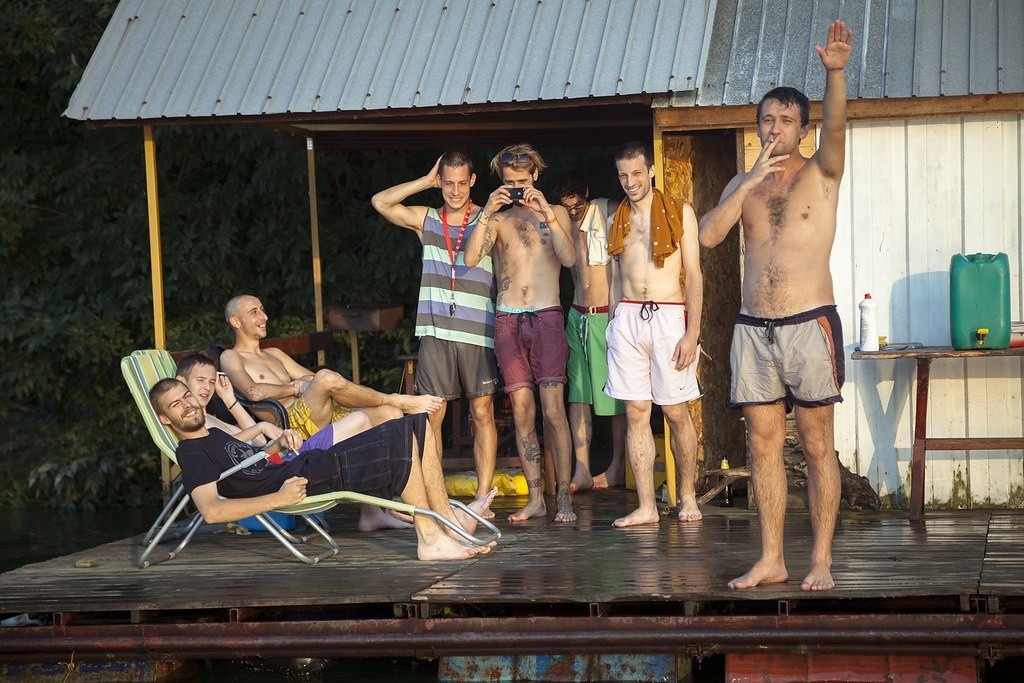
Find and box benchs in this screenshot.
[168,330,339,430]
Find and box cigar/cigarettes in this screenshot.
[218,372,226,375]
[293,448,299,456]
[770,139,773,142]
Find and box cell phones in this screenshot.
[502,187,525,199]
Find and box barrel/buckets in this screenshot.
[950,252,1010,349]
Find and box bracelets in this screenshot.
[478,219,486,225]
[228,400,238,411]
[547,217,556,224]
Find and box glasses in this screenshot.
[501,152,536,165]
[564,199,586,212]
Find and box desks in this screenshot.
[851,346,1024,524]
[400,351,545,469]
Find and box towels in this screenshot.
[608,188,686,268]
[578,197,612,265]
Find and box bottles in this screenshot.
[859,294,879,352]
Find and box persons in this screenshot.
[698,19,850,589]
[219,295,442,522]
[178,353,497,536]
[609,143,703,528]
[372,152,499,518]
[551,170,628,491]
[463,145,576,521]
[152,379,497,560]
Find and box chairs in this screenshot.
[119,348,500,568]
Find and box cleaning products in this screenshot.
[859,292,879,353]
